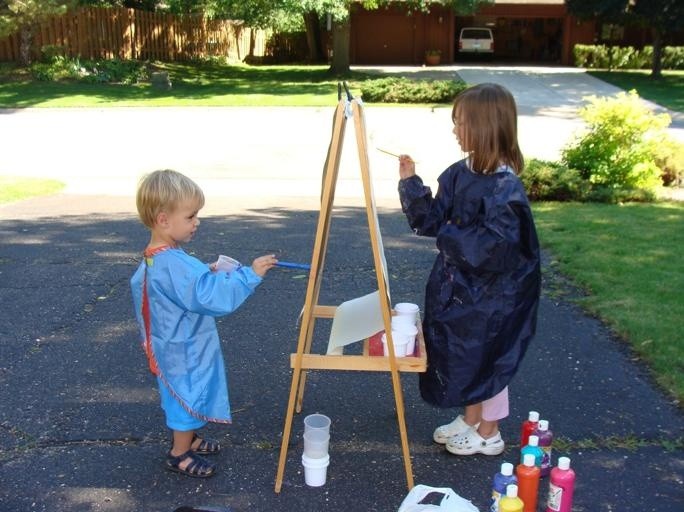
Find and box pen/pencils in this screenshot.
[277,261,311,270]
[378,148,419,166]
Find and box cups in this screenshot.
[213,255,242,272]
[380,302,419,357]
[301,412,333,488]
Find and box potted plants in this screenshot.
[425,48,442,65]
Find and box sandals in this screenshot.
[444,427,506,457]
[432,413,480,444]
[162,445,215,479]
[190,434,221,455]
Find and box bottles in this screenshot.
[489,410,575,512]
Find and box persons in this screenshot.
[397,82,542,457]
[129,169,278,479]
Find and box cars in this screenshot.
[458,27,495,59]
